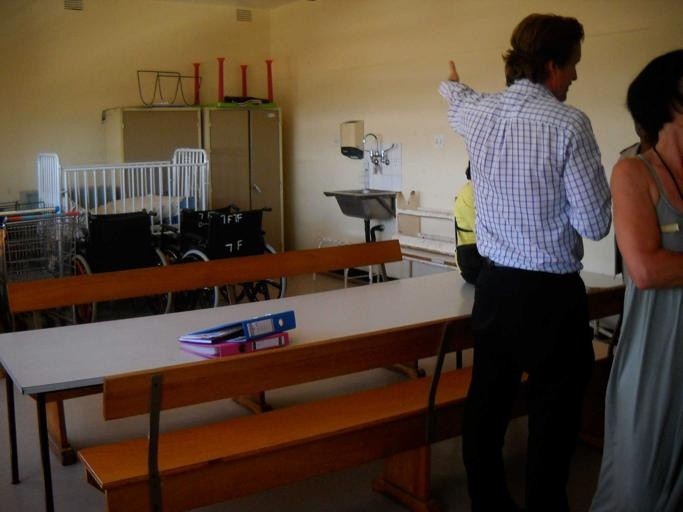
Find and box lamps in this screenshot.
[359,132,396,169]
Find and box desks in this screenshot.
[1,270,629,510]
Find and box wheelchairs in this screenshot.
[54,204,288,328]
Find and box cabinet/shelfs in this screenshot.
[392,190,460,278]
[103,108,286,255]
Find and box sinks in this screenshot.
[323,188,395,220]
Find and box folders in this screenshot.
[176,310,297,360]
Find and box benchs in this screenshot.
[7,241,426,467]
[78,286,628,511]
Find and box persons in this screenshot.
[614,117,653,284]
[586,48,683,512]
[436,12,612,512]
[452,159,481,285]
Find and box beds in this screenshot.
[34,149,210,272]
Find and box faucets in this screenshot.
[362,133,379,158]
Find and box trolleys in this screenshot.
[0,200,81,331]
